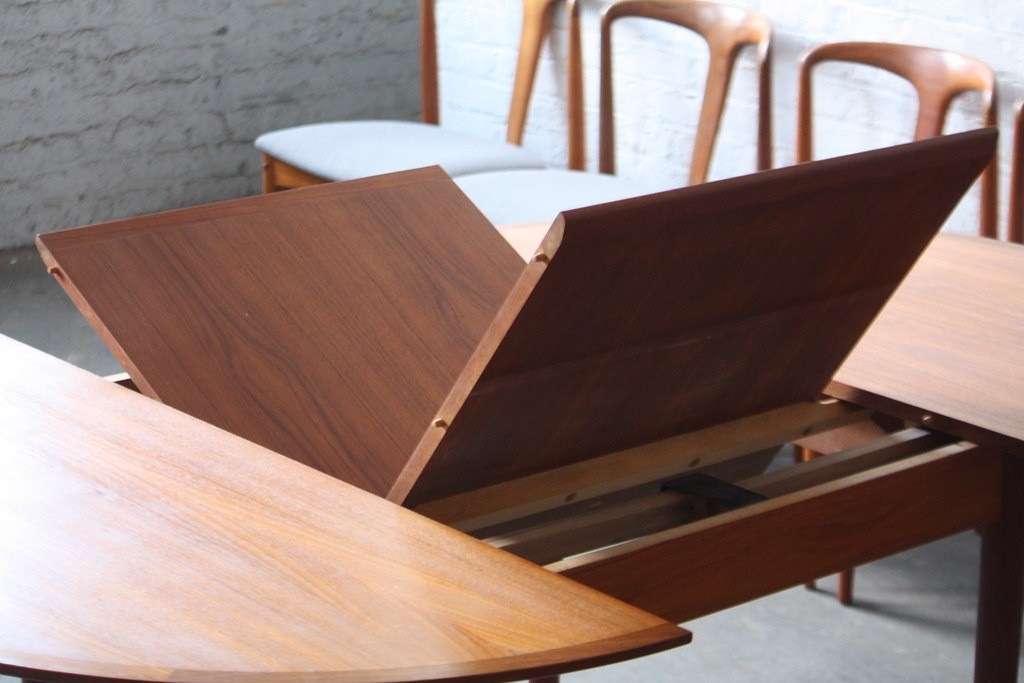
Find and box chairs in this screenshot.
[794,44,1024,247]
[248,0,580,195]
[450,1,778,264]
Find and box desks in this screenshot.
[0,129,1024,682]
